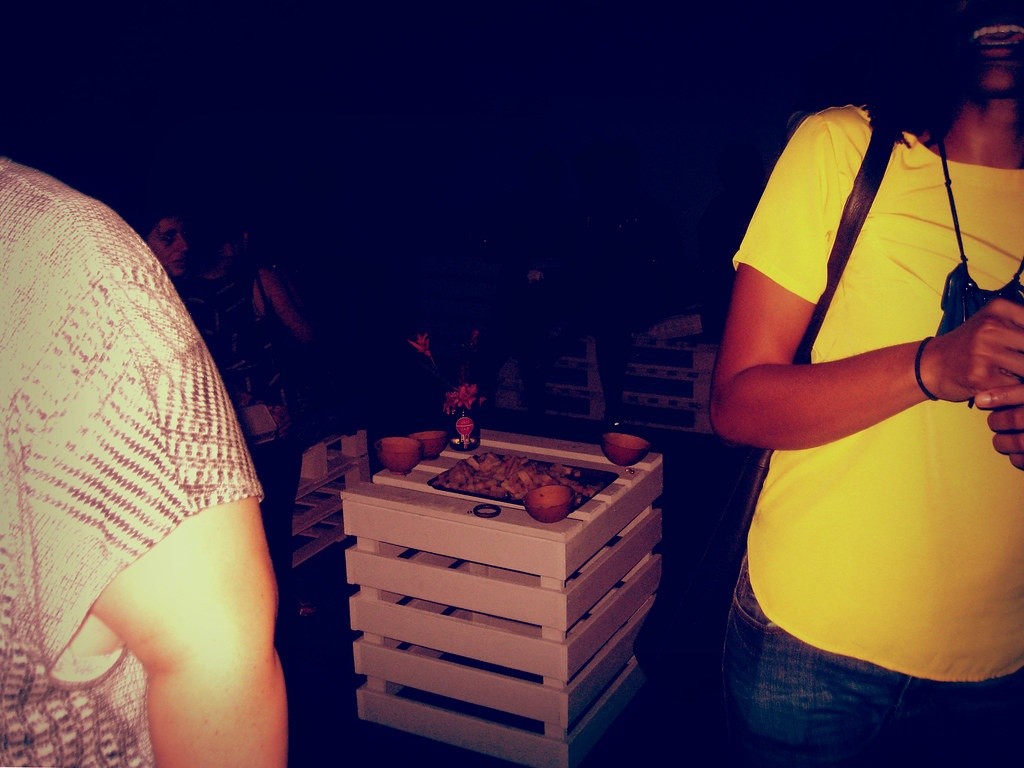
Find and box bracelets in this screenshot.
[474,504,501,517]
[914,337,938,402]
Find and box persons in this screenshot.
[710,1,1024,767]
[0,157,291,767]
[131,196,313,686]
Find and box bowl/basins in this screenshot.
[375,430,447,475]
[524,486,573,524]
[600,433,650,466]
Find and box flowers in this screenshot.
[407,330,487,414]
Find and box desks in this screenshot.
[290,431,369,570]
[340,427,663,768]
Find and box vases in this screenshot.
[447,409,482,451]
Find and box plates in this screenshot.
[427,454,619,518]
